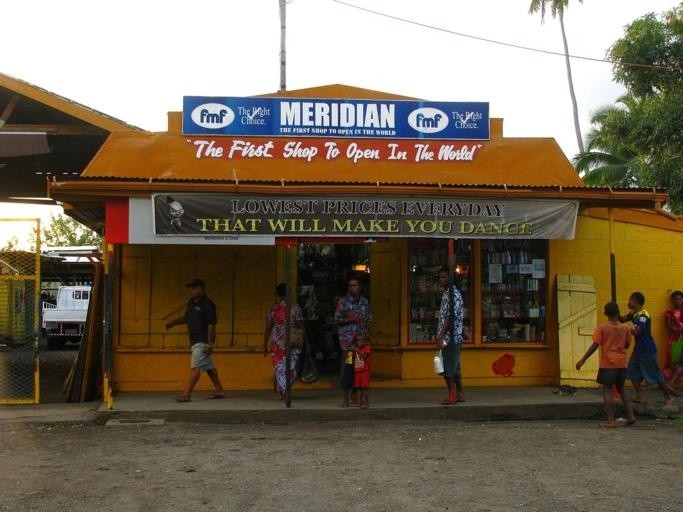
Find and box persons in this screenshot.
[262,282,306,400]
[166,278,226,403]
[299,268,322,384]
[333,277,373,408]
[563,291,683,429]
[435,267,466,404]
[345,335,373,409]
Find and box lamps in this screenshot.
[351,257,366,270]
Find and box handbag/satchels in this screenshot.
[284,327,304,349]
[671,341,683,365]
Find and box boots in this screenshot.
[442,372,465,405]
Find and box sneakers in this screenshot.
[276,390,287,400]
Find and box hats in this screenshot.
[187,280,206,287]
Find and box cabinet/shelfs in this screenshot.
[409,245,471,342]
[483,249,545,342]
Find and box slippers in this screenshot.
[204,393,226,399]
[176,395,192,401]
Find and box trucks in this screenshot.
[41,284,91,348]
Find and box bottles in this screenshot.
[486,244,528,264]
[540,304,545,317]
[530,326,536,341]
[434,356,444,374]
